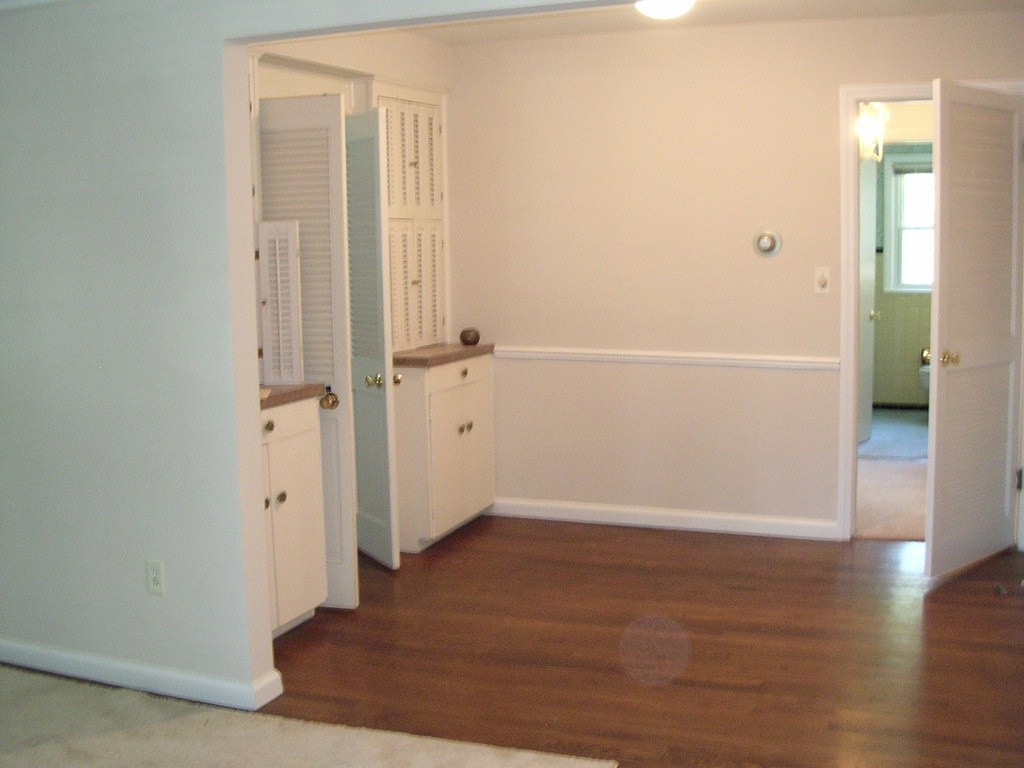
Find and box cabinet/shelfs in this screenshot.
[260,397,329,641]
[397,352,497,554]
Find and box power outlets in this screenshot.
[145,559,163,593]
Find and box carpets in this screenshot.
[854,453,927,543]
[0,663,621,768]
